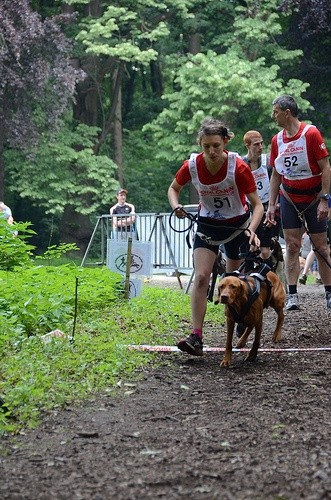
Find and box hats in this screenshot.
[116,187,128,195]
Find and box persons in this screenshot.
[0,200,18,239]
[241,130,284,272]
[168,120,264,356]
[267,95,331,310]
[110,188,139,241]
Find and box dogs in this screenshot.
[214,271,286,366]
[207,250,226,302]
[254,234,312,294]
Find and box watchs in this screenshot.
[321,194,330,200]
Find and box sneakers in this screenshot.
[299,273,307,285]
[236,323,247,338]
[315,277,323,284]
[178,333,204,356]
[325,290,331,308]
[285,291,300,310]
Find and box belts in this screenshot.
[282,183,322,196]
